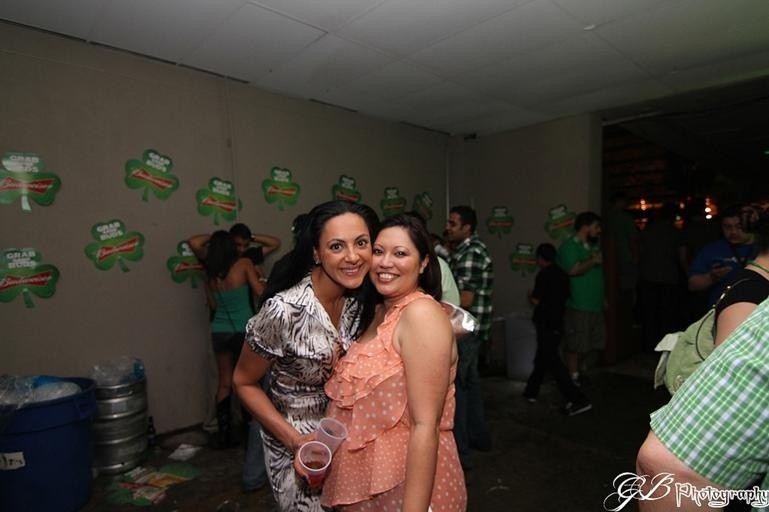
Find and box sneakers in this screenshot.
[523,392,536,403]
[203,415,219,433]
[567,400,592,417]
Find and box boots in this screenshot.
[215,393,240,450]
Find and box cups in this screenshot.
[296,417,348,488]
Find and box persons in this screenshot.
[295,212,468,511]
[634,294,769,511]
[230,198,384,512]
[188,201,769,458]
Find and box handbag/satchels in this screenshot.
[664,308,716,395]
